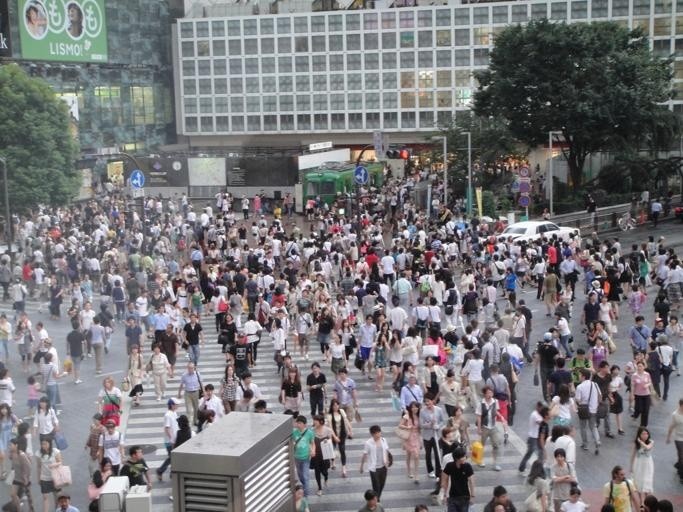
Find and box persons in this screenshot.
[68,4,83,37]
[26,7,48,37]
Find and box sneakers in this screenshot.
[315,464,347,496]
[490,463,527,479]
[579,429,626,455]
[155,468,163,481]
[131,393,166,409]
[72,370,102,384]
[407,469,442,484]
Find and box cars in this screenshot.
[495,220,582,247]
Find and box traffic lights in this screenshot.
[386,148,410,160]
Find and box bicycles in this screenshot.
[617,211,638,232]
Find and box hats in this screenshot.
[592,280,601,288]
[93,413,115,428]
[56,493,71,500]
[654,318,664,324]
[542,325,560,342]
[176,414,190,427]
[658,334,668,343]
[165,397,182,405]
[447,325,456,333]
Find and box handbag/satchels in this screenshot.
[532,369,540,387]
[620,271,630,283]
[49,466,72,489]
[444,305,454,315]
[422,344,439,358]
[415,318,433,331]
[470,441,484,466]
[62,353,74,376]
[198,388,204,399]
[352,406,362,423]
[350,335,363,370]
[498,268,506,275]
[54,433,70,452]
[217,330,228,345]
[660,363,673,375]
[121,375,131,392]
[218,299,230,312]
[146,363,154,372]
[445,289,458,305]
[524,491,544,512]
[400,346,417,357]
[570,480,578,488]
[507,406,516,427]
[319,438,335,460]
[420,275,432,294]
[393,426,412,440]
[577,402,609,419]
[181,340,189,351]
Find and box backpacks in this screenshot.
[289,254,302,271]
[593,289,604,303]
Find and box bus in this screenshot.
[301,158,386,216]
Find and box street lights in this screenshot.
[549,130,564,216]
[80,152,147,254]
[460,132,474,219]
[431,135,449,208]
